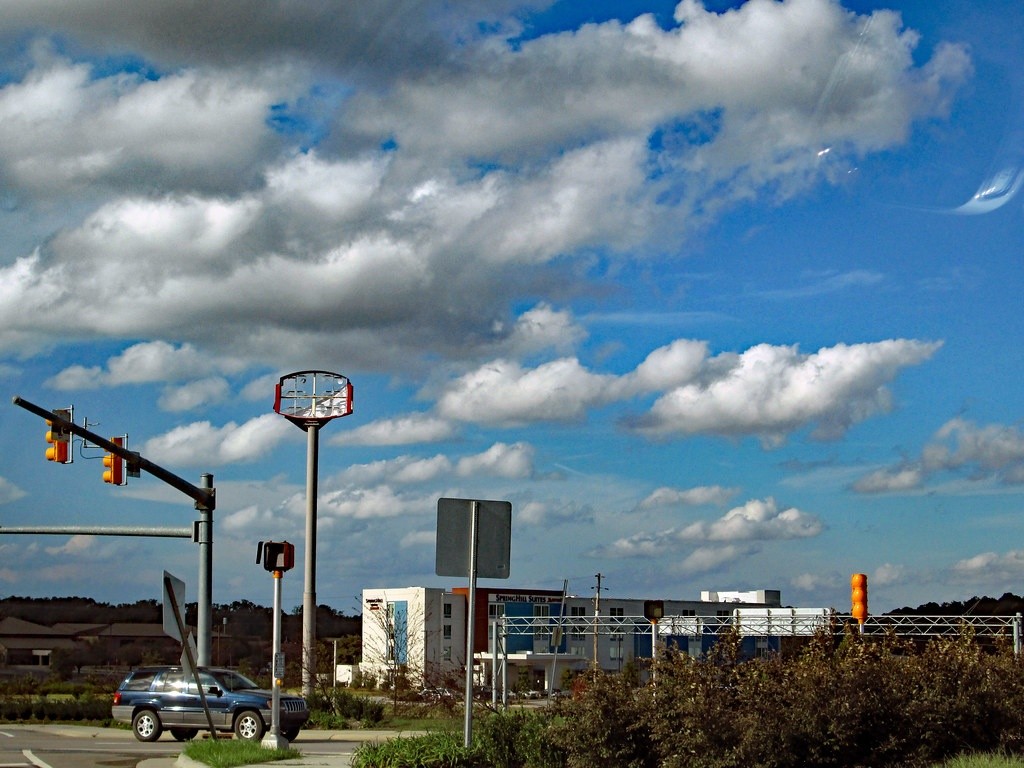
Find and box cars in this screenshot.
[475,686,573,701]
[416,687,465,702]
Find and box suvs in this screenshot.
[111,664,311,743]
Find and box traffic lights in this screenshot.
[643,600,664,624]
[45,409,72,463]
[262,541,290,571]
[101,438,122,486]
